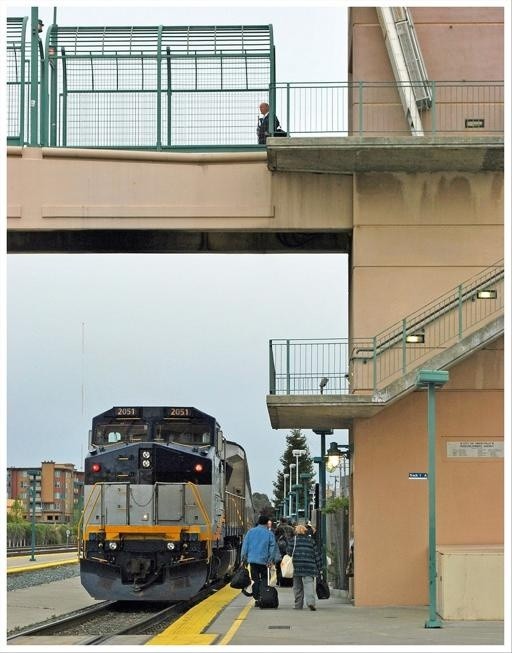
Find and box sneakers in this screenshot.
[308,604,317,611]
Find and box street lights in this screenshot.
[319,376,329,581]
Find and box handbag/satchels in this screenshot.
[231,569,250,589]
[316,573,330,599]
[279,555,293,587]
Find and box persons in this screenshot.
[240,515,276,586]
[275,516,293,541]
[305,524,313,535]
[256,101,280,144]
[288,524,322,610]
[267,519,275,532]
[289,522,292,525]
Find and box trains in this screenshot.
[76,405,257,602]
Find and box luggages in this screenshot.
[259,567,279,609]
